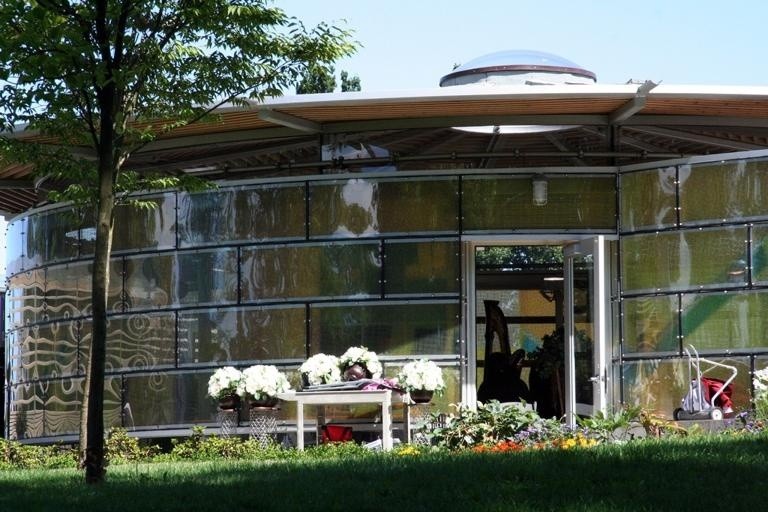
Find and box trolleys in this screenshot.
[673,343,738,420]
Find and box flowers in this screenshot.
[397,356,449,397]
[209,343,384,401]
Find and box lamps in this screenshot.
[531,173,551,206]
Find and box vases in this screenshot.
[217,393,278,408]
[408,388,434,403]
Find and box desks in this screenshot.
[295,390,411,457]
